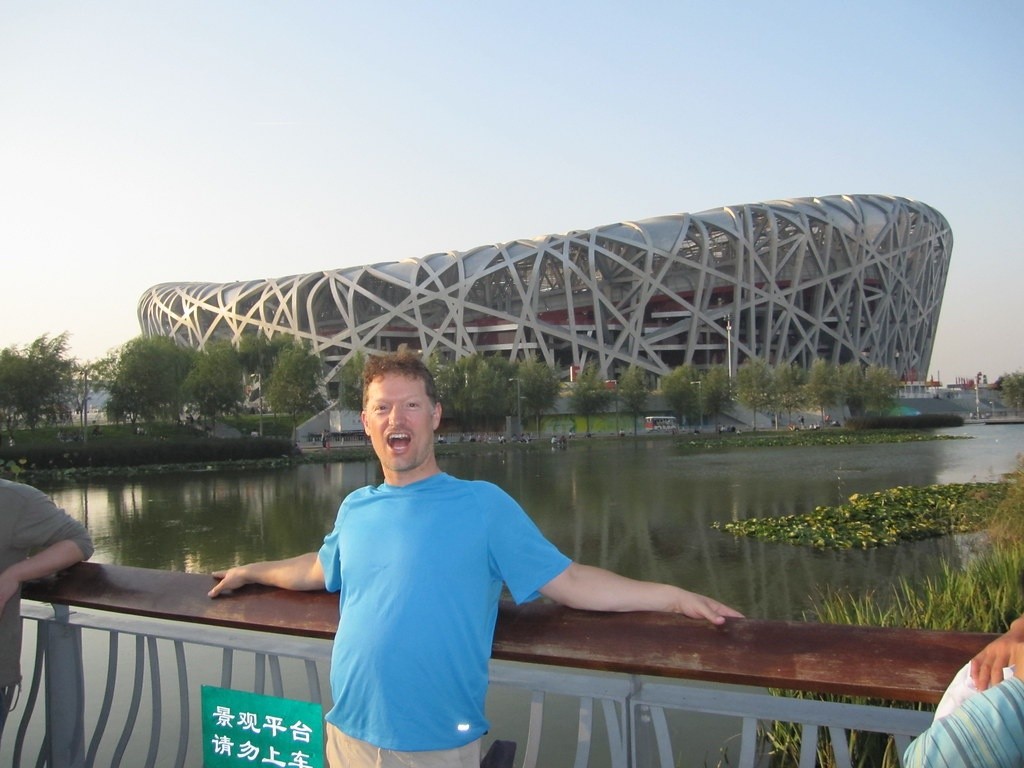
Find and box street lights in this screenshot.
[250,374,264,438]
[722,313,733,396]
[690,381,703,430]
[509,378,521,433]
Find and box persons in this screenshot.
[438,430,591,446]
[0,479,95,741]
[184,404,272,438]
[970,412,990,419]
[207,352,745,768]
[57,420,148,442]
[619,419,741,437]
[903,613,1024,768]
[789,415,830,431]
[321,428,331,448]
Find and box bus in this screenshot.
[645,416,677,431]
